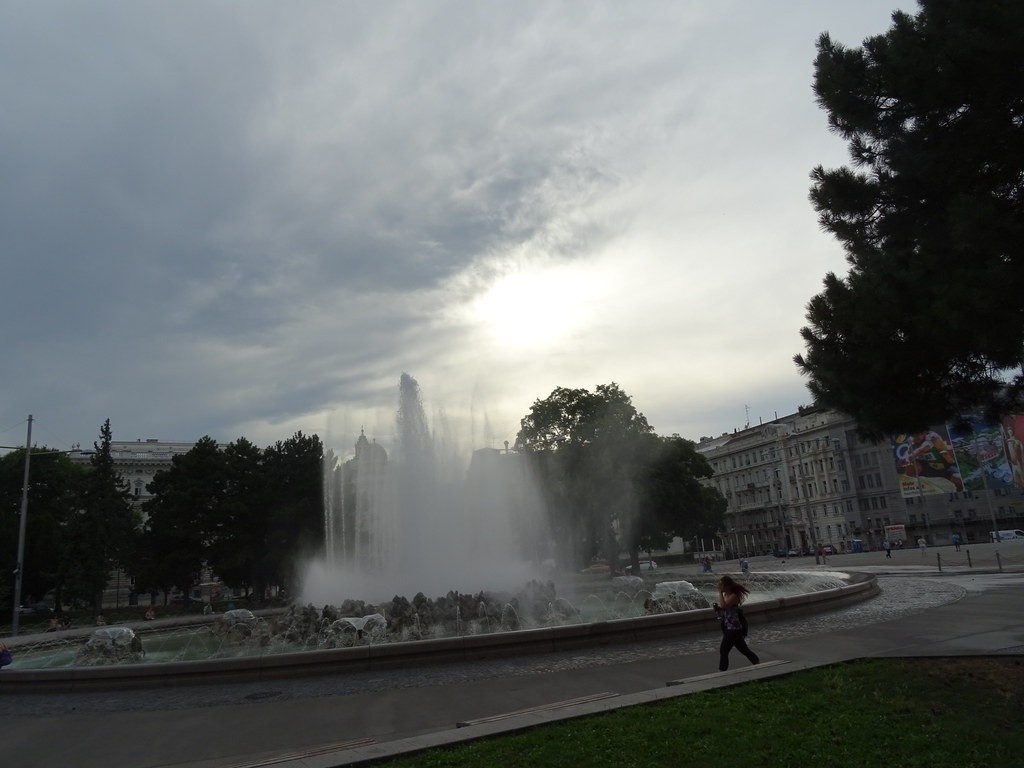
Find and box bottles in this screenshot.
[713,603,723,620]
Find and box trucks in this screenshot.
[988,528,1024,543]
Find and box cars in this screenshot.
[626,560,658,572]
[788,544,838,557]
[580,564,611,575]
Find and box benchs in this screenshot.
[42,626,78,632]
[92,622,123,627]
[0,630,33,638]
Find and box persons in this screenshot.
[144,607,156,619]
[895,429,964,492]
[821,547,826,565]
[0,640,12,668]
[952,530,960,552]
[48,614,62,632]
[96,612,106,627]
[203,602,212,615]
[714,575,760,672]
[917,536,927,558]
[885,539,892,559]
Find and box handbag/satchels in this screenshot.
[721,608,748,636]
